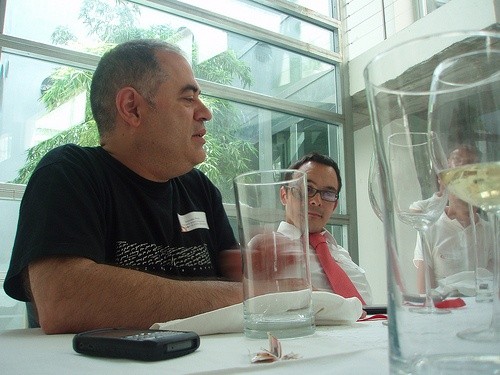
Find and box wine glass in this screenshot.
[368,131,451,315]
[427,49,500,344]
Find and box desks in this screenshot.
[0,296,500,375]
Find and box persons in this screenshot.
[411,145,500,294]
[273,152,372,305]
[3,38,319,335]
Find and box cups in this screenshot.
[233,168,315,339]
[363,29,500,373]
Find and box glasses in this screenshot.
[307,185,339,202]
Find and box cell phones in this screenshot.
[72,327,200,361]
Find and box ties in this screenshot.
[309,234,366,305]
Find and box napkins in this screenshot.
[149,290,363,335]
